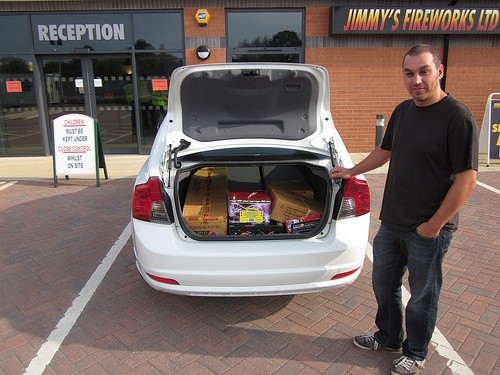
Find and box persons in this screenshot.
[330,44,479,375]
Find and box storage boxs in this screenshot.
[264,182,324,223]
[182,167,228,235]
[228,190,272,225]
[271,179,314,200]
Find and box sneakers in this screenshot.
[353,332,403,352]
[390,354,426,375]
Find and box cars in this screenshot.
[129,61,371,298]
